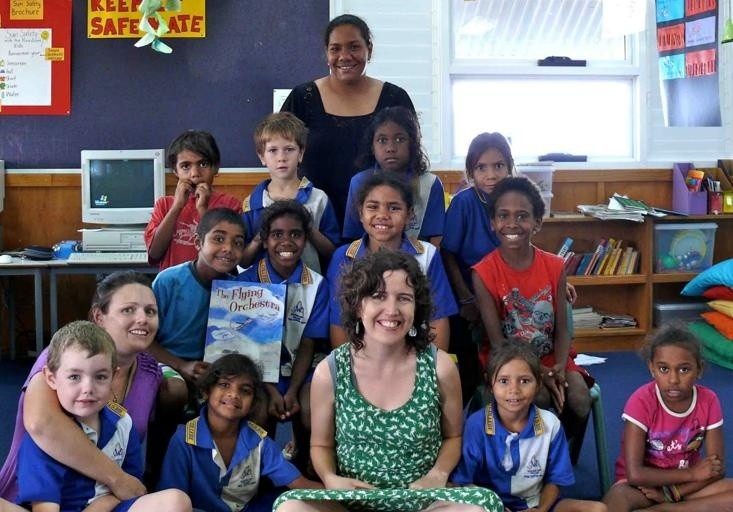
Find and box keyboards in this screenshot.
[67,252,149,263]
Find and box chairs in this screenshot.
[466,298,611,495]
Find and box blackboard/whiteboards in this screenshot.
[0,0,336,173]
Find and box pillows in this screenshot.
[680,259,733,368]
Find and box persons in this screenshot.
[338,106,444,262]
[0,168,606,511]
[240,109,344,284]
[144,126,248,279]
[599,324,732,511]
[438,132,578,412]
[276,13,421,242]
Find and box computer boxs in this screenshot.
[82,231,147,251]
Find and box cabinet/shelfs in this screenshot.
[532,214,733,353]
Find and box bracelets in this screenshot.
[662,485,681,503]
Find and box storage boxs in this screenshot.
[654,222,718,273]
[515,166,554,217]
[652,299,707,328]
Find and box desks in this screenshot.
[0,252,159,359]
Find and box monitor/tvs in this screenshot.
[81,148,166,231]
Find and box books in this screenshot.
[557,234,641,276]
[570,302,638,329]
[550,192,688,222]
